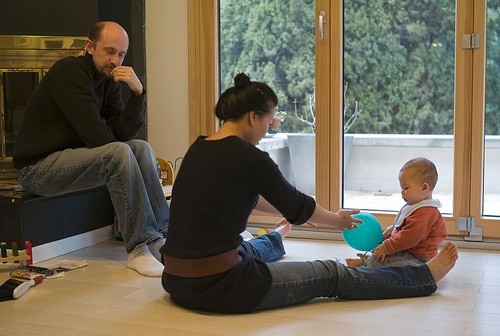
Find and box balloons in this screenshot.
[343,211,384,251]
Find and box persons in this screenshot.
[345,156,448,269]
[12,21,174,278]
[159,72,459,316]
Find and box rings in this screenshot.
[348,223,354,227]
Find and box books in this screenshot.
[9,258,89,280]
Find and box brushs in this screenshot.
[0,275,44,302]
